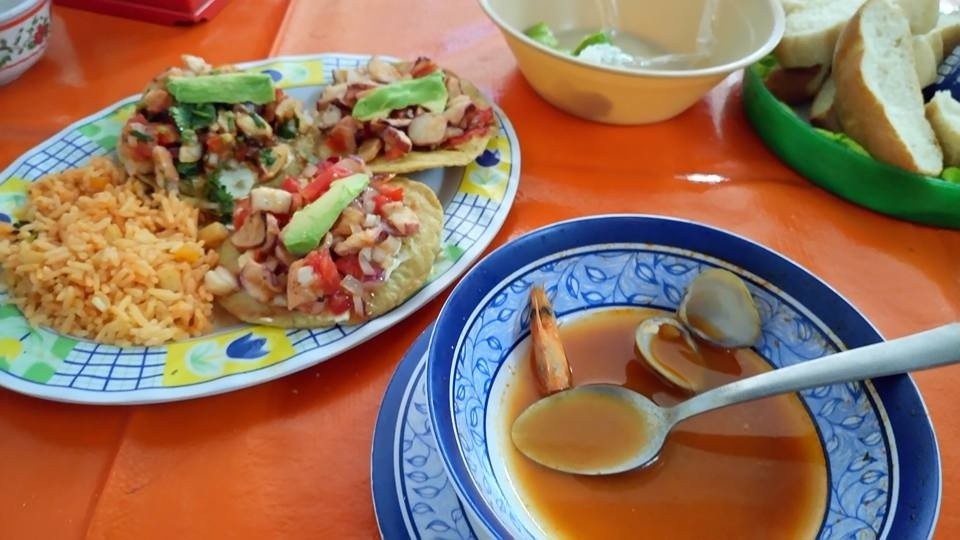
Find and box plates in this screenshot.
[0,53,521,406]
[371,317,476,539]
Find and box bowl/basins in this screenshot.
[476,1,786,126]
[743,51,960,231]
[424,213,943,539]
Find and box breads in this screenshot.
[764,0,960,182]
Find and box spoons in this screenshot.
[510,322,960,475]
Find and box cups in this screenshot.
[0,0,51,85]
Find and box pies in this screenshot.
[114,60,497,329]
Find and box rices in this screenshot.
[0,154,218,345]
